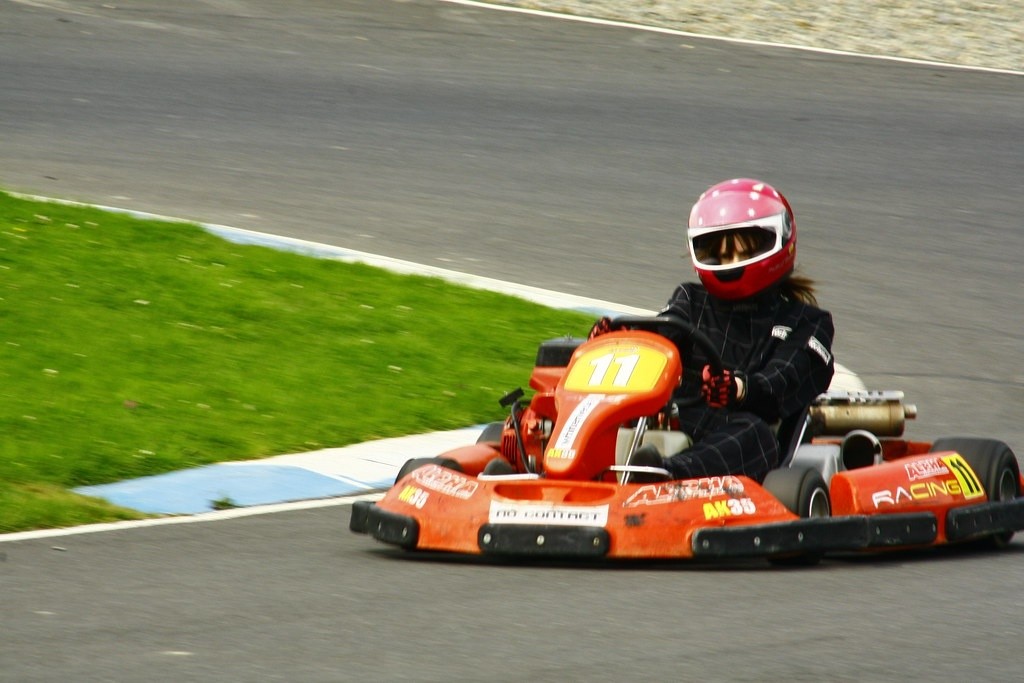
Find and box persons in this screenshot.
[589,178,836,486]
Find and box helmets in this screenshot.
[687,178,797,300]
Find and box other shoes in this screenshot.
[632,446,671,482]
[484,458,513,475]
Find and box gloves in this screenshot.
[588,317,630,338]
[692,366,737,410]
